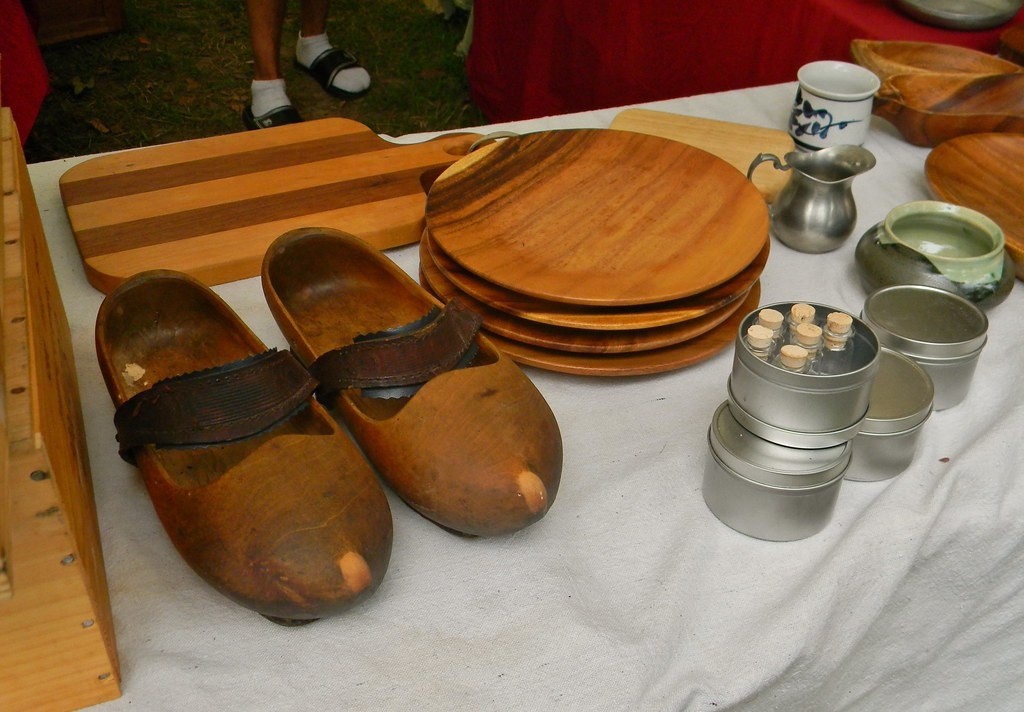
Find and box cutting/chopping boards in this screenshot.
[59,118,497,296]
[610,109,795,206]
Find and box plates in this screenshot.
[420,128,770,377]
[924,134,1023,283]
[893,0,1022,30]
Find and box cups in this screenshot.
[788,61,881,153]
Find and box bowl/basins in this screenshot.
[873,73,1024,148]
[851,39,1024,84]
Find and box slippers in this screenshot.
[292,47,371,100]
[242,105,304,131]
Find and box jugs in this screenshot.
[747,145,876,253]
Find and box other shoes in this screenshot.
[92,267,394,624]
[262,225,564,542]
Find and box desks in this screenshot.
[468,0,1024,124]
[23,83,1024,712]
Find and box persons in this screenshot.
[247,1,373,131]
